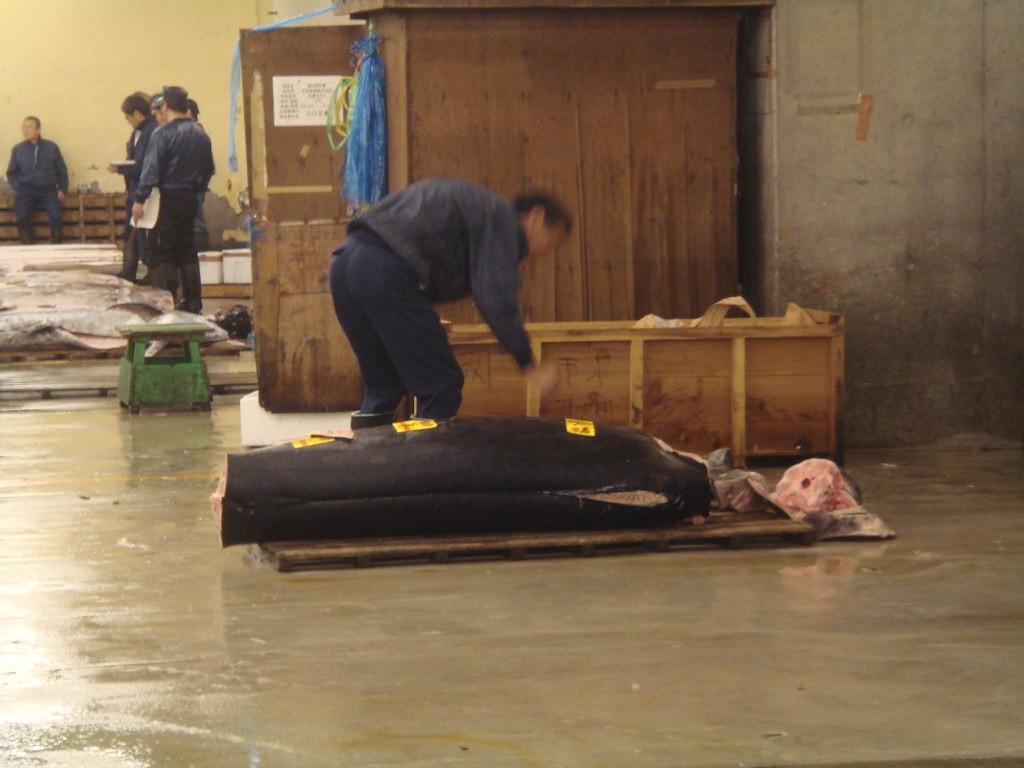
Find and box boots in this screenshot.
[176,258,203,311]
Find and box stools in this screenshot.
[116,322,214,415]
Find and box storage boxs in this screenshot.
[223,248,252,284]
[198,250,223,285]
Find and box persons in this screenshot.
[330,179,572,426]
[6,117,69,244]
[106,87,215,313]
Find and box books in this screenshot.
[110,160,135,166]
